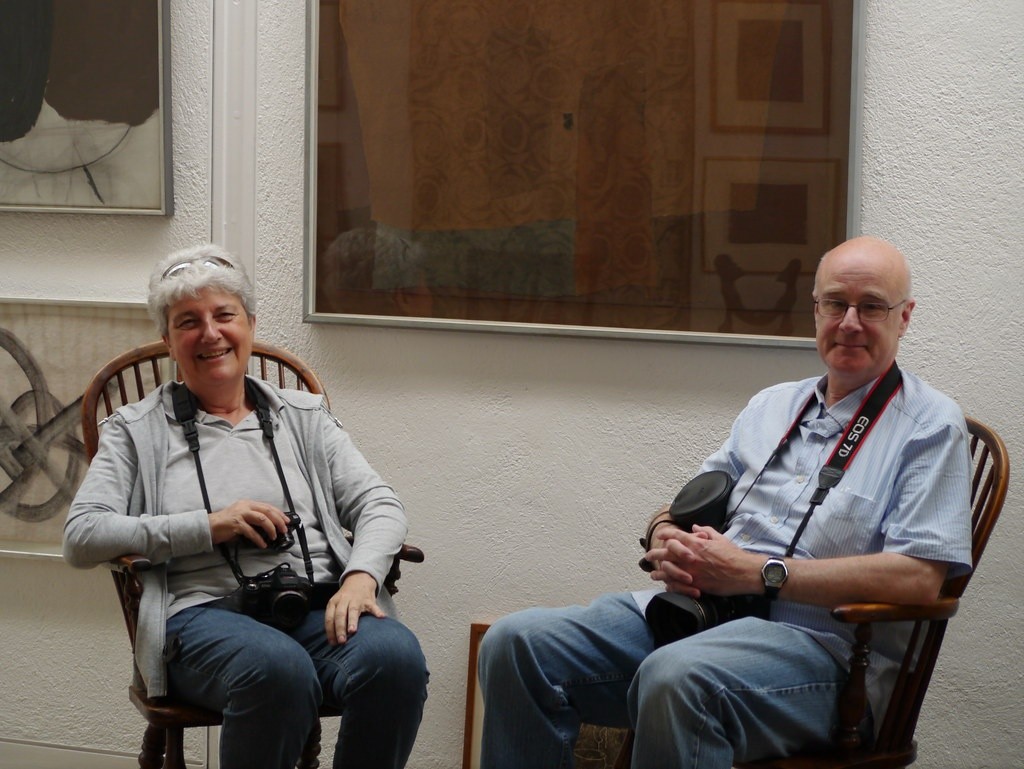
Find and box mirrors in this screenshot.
[303,0,867,350]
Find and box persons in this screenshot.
[63,244,431,769]
[477,236,974,769]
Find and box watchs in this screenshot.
[761,556,789,600]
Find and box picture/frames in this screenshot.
[0,297,178,565]
[0,0,175,216]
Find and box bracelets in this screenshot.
[646,520,676,553]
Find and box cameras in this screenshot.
[642,584,757,655]
[232,563,316,633]
[229,510,302,557]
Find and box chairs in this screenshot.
[612,416,1011,769]
[81,341,432,769]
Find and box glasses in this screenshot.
[813,298,906,323]
[161,256,234,281]
